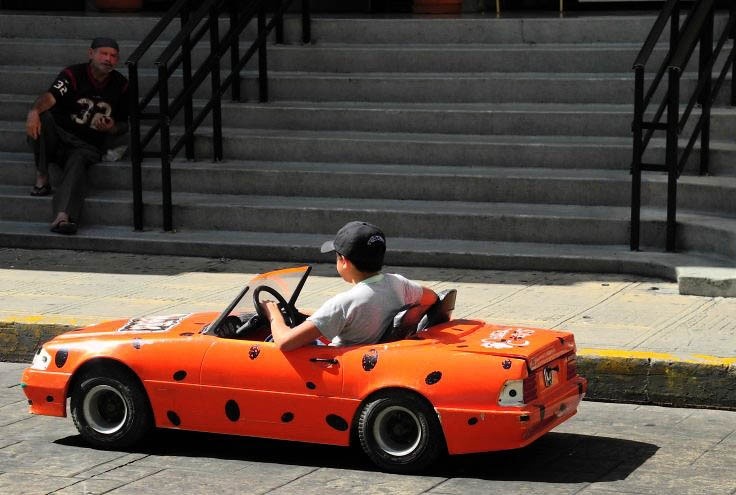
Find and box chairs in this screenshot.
[416,288,457,333]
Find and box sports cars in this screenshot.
[19,265,589,477]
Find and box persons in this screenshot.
[258,221,438,352]
[24,37,131,233]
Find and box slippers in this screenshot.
[52,220,77,234]
[29,185,48,197]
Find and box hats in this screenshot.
[91,37,119,51]
[320,220,387,259]
